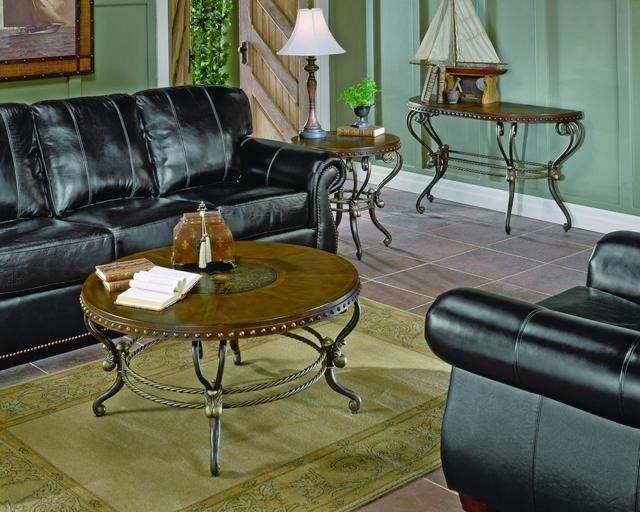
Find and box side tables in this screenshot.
[291,133,403,261]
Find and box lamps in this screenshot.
[275,0,347,139]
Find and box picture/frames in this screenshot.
[0,0,94,83]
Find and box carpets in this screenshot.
[0,297,452,512]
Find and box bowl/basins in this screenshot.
[444,90,460,104]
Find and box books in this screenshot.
[438,64,446,104]
[336,122,385,138]
[420,65,439,103]
[102,278,135,293]
[94,257,157,282]
[113,265,203,313]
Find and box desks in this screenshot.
[404,95,584,236]
[79,240,364,476]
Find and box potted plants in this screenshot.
[337,74,382,128]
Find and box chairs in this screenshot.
[423,230,640,512]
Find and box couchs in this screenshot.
[0,85,348,374]
